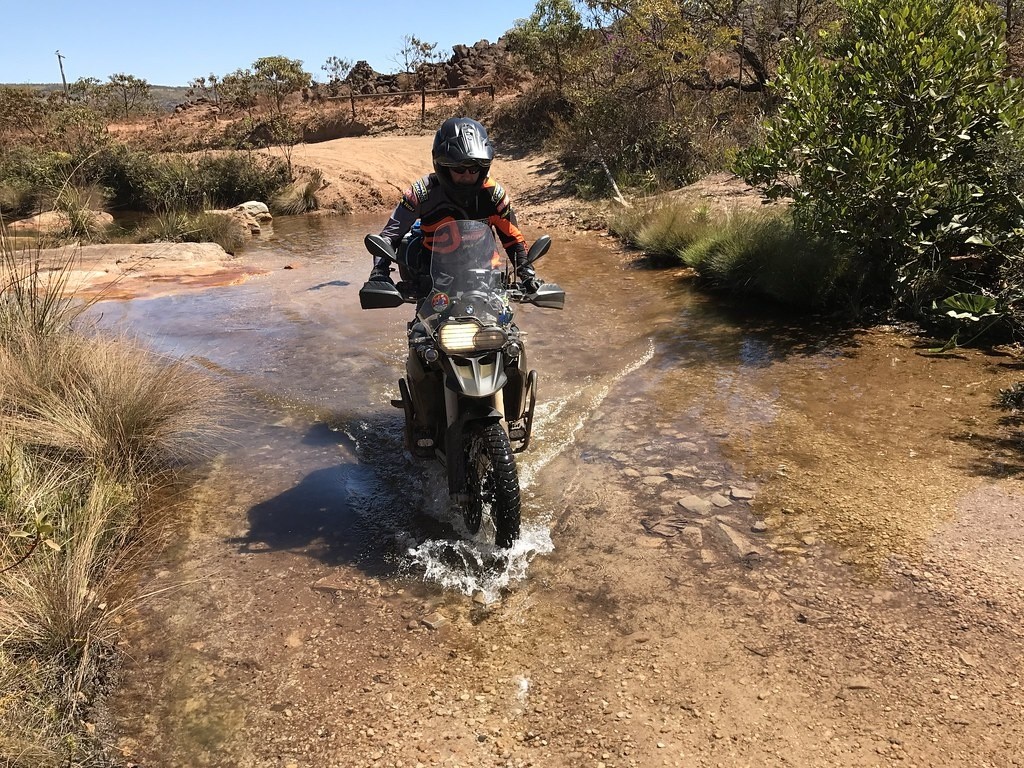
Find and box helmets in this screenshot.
[432,116,495,194]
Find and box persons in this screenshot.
[365,115,546,354]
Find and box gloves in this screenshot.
[523,275,546,293]
[370,265,394,284]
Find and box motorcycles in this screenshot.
[358,233,566,566]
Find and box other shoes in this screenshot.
[413,427,434,454]
[508,419,527,439]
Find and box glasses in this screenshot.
[451,163,483,176]
